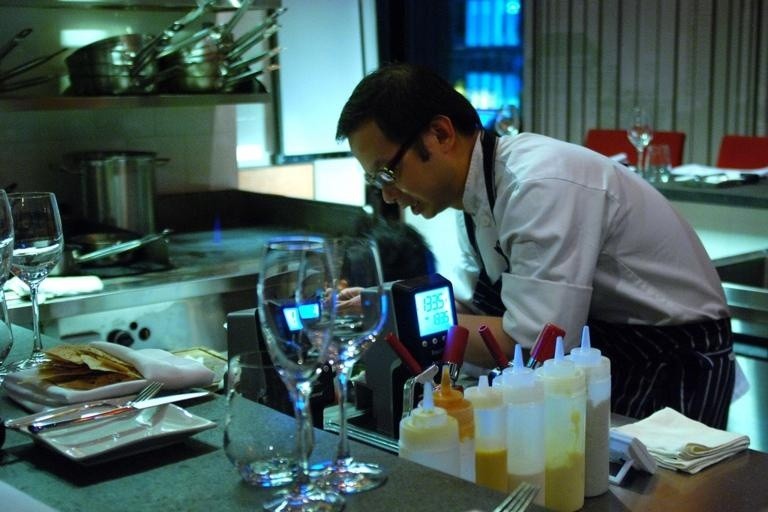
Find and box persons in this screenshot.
[335,65,736,431]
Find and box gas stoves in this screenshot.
[3,240,231,350]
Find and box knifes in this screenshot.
[25,389,210,437]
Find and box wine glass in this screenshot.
[299,233,392,496]
[256,238,348,512]
[626,105,655,181]
[0,188,66,374]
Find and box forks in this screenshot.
[486,482,541,510]
[4,378,165,431]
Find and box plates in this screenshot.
[2,397,216,466]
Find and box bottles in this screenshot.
[462,374,509,496]
[566,325,610,498]
[491,343,547,511]
[532,334,586,510]
[434,364,476,484]
[398,382,460,481]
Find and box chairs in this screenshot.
[584,129,767,170]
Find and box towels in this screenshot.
[611,406,753,477]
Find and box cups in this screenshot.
[223,348,306,490]
[645,143,673,184]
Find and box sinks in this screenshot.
[711,246,768,341]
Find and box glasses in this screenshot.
[365,141,411,189]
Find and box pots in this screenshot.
[64,1,287,96]
[46,146,174,236]
